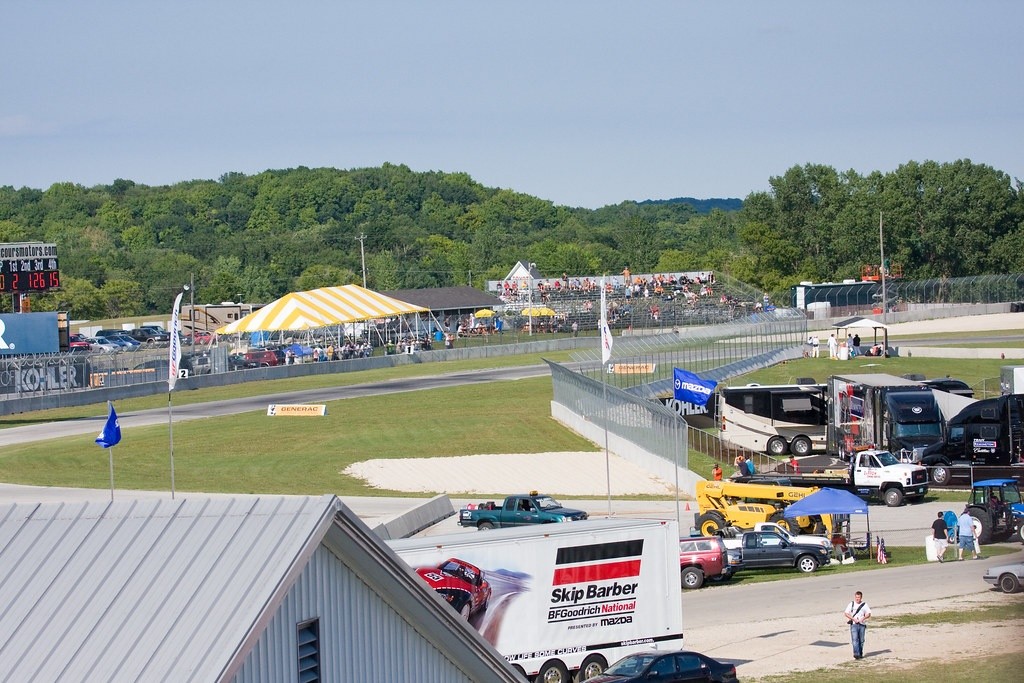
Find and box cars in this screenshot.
[578,648,740,683]
[983,558,1024,593]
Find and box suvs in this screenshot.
[679,536,729,589]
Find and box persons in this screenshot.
[847,334,861,356]
[653,310,658,319]
[932,512,949,563]
[808,335,819,357]
[789,456,799,473]
[444,313,504,349]
[844,591,871,659]
[598,318,601,336]
[827,334,837,359]
[540,320,546,325]
[387,338,435,355]
[712,462,722,480]
[800,524,815,534]
[957,508,980,561]
[673,322,679,333]
[285,342,374,365]
[619,266,630,288]
[736,454,755,476]
[872,342,884,356]
[832,534,856,558]
[496,270,776,312]
[572,321,578,338]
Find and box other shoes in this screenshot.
[973,555,980,560]
[853,655,864,659]
[959,558,964,561]
[937,554,943,563]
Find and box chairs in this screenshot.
[521,500,532,511]
[834,544,851,561]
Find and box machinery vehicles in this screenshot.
[692,479,847,543]
[963,478,1024,545]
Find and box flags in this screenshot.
[599,279,613,364]
[95,402,121,448]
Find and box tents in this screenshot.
[784,487,872,563]
[831,316,890,358]
[475,308,496,331]
[208,283,431,362]
[522,308,555,332]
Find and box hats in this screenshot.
[789,455,794,458]
[714,464,718,468]
[745,455,749,460]
[963,508,970,513]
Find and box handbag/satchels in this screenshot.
[974,538,981,554]
[847,620,853,625]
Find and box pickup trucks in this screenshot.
[725,531,831,574]
[458,491,588,531]
[712,522,835,552]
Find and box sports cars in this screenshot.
[414,558,491,624]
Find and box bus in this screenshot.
[717,378,972,457]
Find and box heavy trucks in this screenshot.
[733,446,929,508]
[921,393,1024,487]
[381,517,685,683]
[833,372,945,460]
